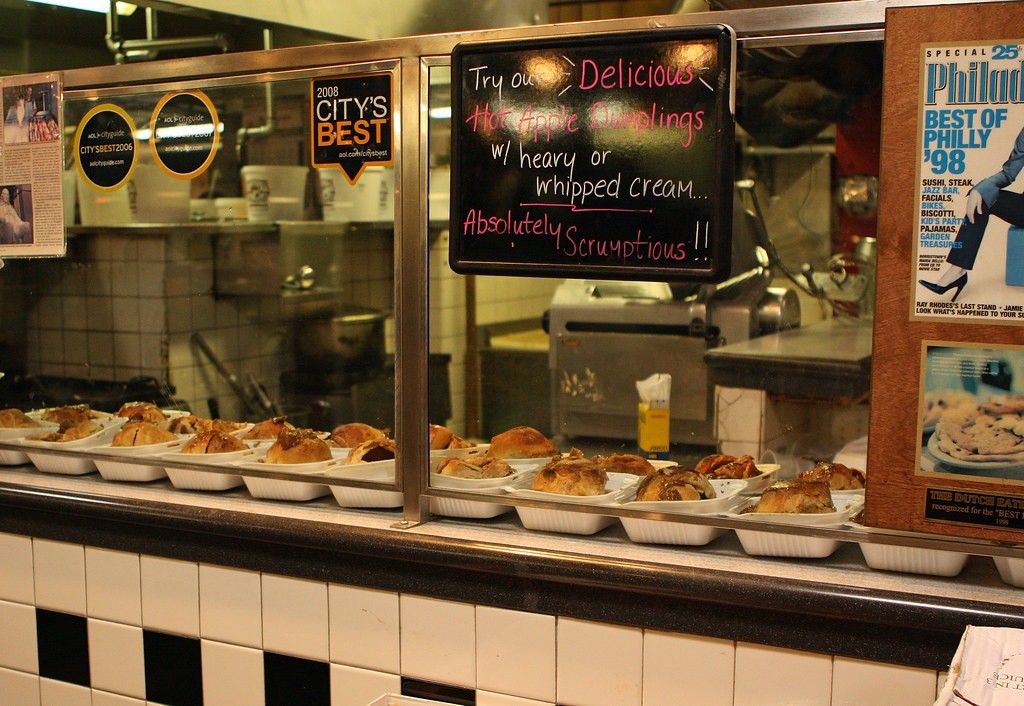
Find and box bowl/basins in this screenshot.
[0,403,1024,588]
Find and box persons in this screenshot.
[0,188,31,244]
[5,87,59,142]
[669,0,883,149]
[919,126,1024,302]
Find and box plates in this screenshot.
[927,431,1024,469]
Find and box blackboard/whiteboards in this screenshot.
[448,23,737,283]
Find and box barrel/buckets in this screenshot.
[64,164,192,226]
[317,166,385,222]
[241,163,310,225]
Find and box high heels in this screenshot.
[918,272,968,302]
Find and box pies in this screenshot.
[914,388,1024,463]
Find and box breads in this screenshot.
[0,400,866,517]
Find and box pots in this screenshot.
[281,304,393,372]
